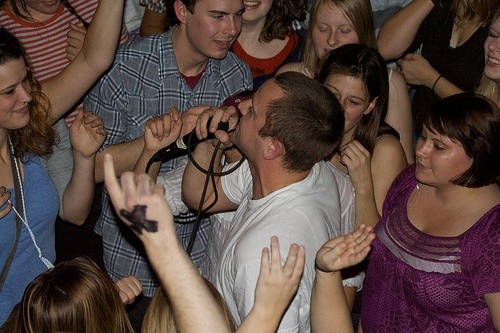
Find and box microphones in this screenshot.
[153,116,229,162]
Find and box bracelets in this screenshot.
[315,257,334,273]
[432,74,442,91]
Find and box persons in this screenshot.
[0,0,500,333]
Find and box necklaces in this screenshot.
[3,135,55,269]
